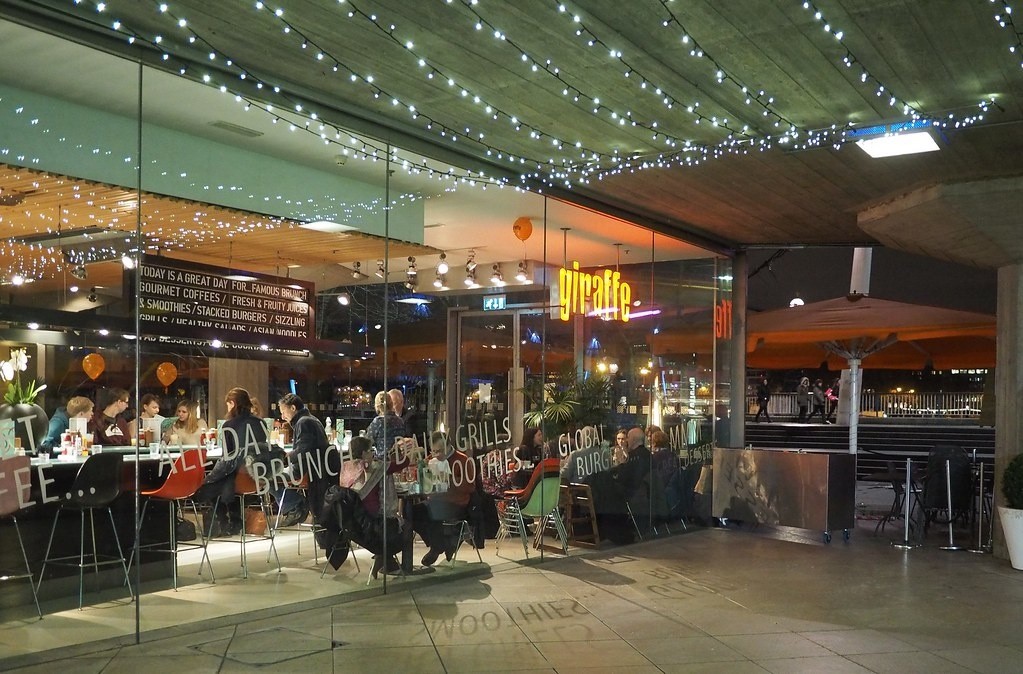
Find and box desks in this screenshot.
[378,481,437,574]
[861,473,993,514]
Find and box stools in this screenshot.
[0,444,341,621]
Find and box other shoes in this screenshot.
[422,544,445,565]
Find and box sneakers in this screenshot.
[298,501,310,524]
[281,507,303,526]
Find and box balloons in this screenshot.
[157,362,178,386]
[513,218,533,241]
[83,352,105,380]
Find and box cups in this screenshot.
[206,441,215,454]
[60,429,102,455]
[326,433,333,445]
[149,443,159,458]
[270,431,279,444]
[359,429,366,436]
[344,429,352,442]
[509,455,540,470]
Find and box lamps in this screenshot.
[68,268,88,279]
[122,254,136,269]
[351,249,529,290]
[86,288,98,302]
[314,293,350,305]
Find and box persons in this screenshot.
[366,388,406,461]
[88,388,131,446]
[250,397,263,418]
[796,377,811,424]
[412,432,476,566]
[128,394,167,446]
[825,378,840,425]
[214,388,267,537]
[807,379,829,425]
[755,379,773,423]
[166,401,208,446]
[340,436,413,580]
[39,396,94,459]
[269,393,329,526]
[481,426,679,530]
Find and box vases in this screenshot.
[0,403,49,455]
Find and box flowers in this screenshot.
[0,347,47,407]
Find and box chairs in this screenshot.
[316,453,704,587]
[872,458,993,541]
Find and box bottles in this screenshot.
[138,429,145,446]
[200,428,217,446]
[392,468,415,486]
[324,417,332,433]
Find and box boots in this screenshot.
[229,519,243,535]
[216,521,231,537]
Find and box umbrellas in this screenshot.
[323,312,608,453]
[650,293,998,520]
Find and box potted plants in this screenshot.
[503,359,617,453]
[997,453,1023,571]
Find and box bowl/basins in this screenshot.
[58,455,70,462]
